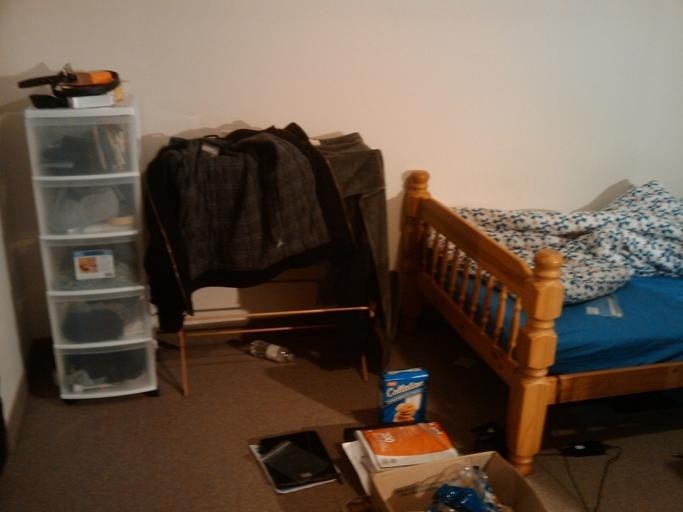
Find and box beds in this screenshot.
[399,170,683,477]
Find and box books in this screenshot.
[260,429,337,490]
[343,420,432,442]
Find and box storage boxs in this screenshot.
[370,451,545,512]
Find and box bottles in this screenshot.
[249,340,295,363]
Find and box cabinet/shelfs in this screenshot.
[23,105,160,403]
